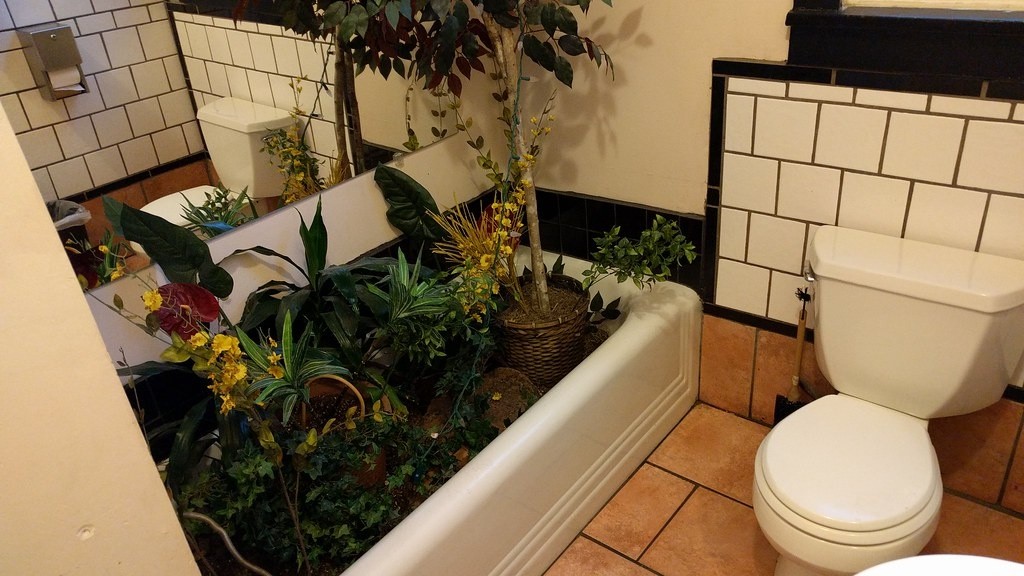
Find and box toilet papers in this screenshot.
[47,64,84,91]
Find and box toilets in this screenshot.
[752,224,1018,574]
[130,96,299,261]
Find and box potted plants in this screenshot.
[290,380,460,573]
[319,0,699,391]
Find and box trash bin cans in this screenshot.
[43,199,92,262]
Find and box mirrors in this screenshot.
[0,0,461,297]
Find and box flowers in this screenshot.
[427,84,558,326]
[63,224,128,293]
[257,74,328,204]
[119,265,284,452]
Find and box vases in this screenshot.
[296,373,373,440]
